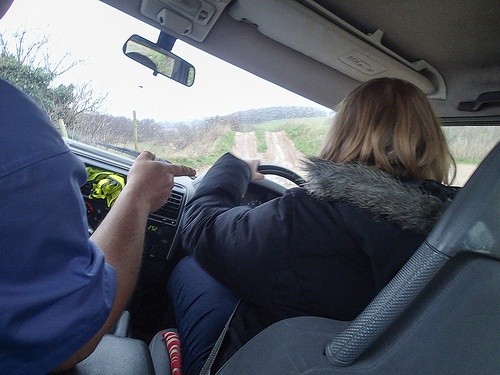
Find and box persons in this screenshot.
[0,0,196,375]
[170,76,456,374]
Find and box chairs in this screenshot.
[152,144,500,375]
[61,335,155,375]
